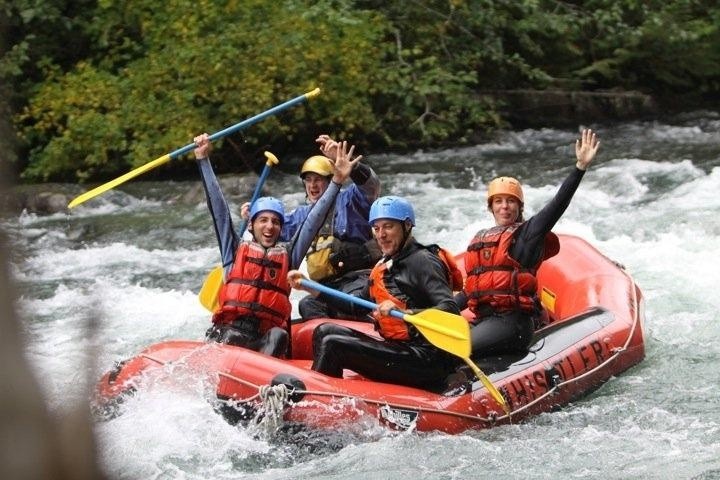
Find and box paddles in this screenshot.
[68,87,321,208]
[294,276,505,407]
[198,150,279,315]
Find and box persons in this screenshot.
[284,194,462,395]
[185,130,364,362]
[238,134,387,322]
[449,127,602,377]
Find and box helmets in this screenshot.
[485,175,525,208]
[368,195,417,228]
[298,152,336,177]
[247,195,285,230]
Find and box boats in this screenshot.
[97,234,645,437]
